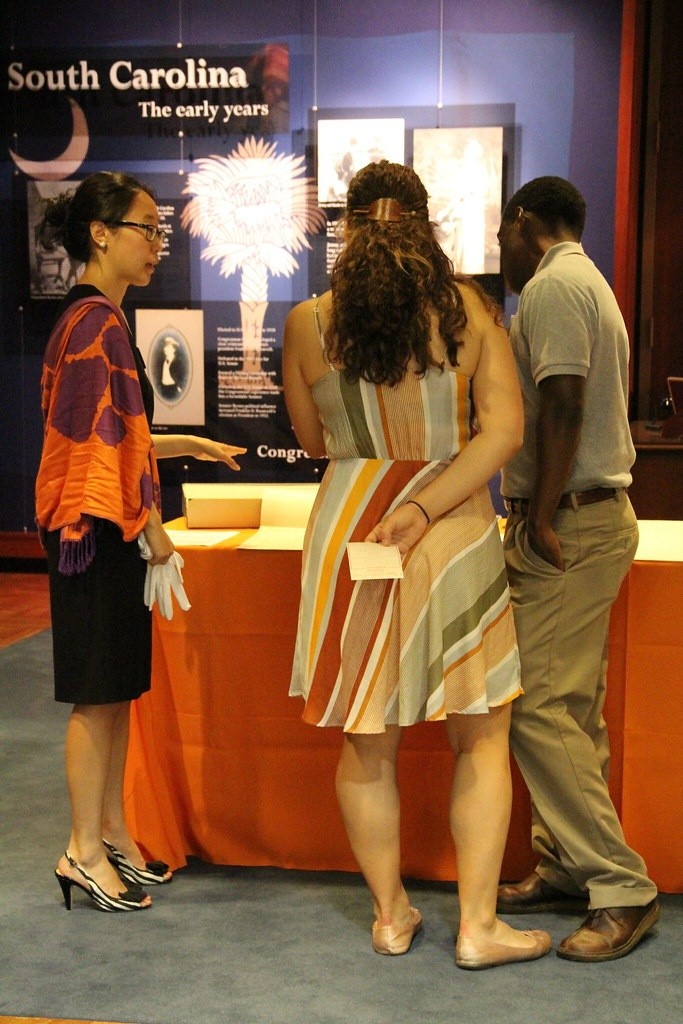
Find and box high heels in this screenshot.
[103,839,171,885]
[55,849,151,912]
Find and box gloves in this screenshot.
[137,534,192,619]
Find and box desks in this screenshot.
[120,483,683,895]
[630,420,683,520]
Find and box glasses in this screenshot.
[110,221,166,242]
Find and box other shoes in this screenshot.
[372,907,423,955]
[456,930,552,969]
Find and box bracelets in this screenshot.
[406,500,430,525]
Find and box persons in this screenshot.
[436,133,499,272]
[31,170,249,915]
[328,149,388,201]
[283,159,554,972]
[474,174,663,964]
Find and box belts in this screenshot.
[502,488,615,514]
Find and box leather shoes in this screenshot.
[496,872,589,912]
[557,897,659,962]
